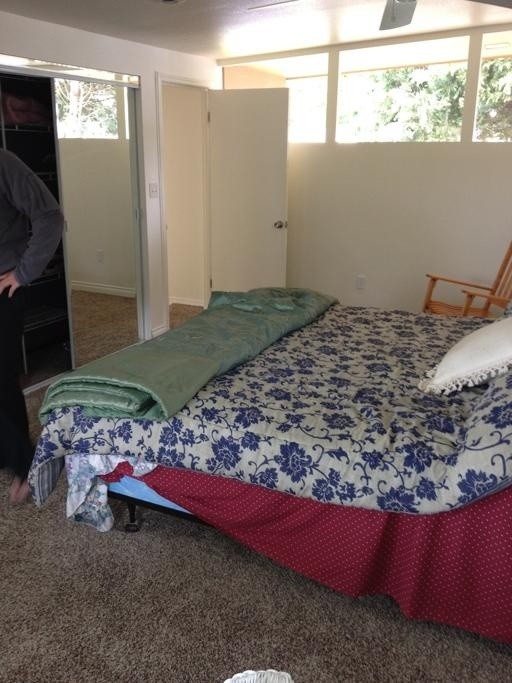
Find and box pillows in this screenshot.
[418,316,512,393]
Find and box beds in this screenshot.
[40,286,512,646]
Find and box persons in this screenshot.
[0,147,64,505]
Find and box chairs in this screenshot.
[422,241,512,316]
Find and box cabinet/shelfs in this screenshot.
[0,64,74,393]
